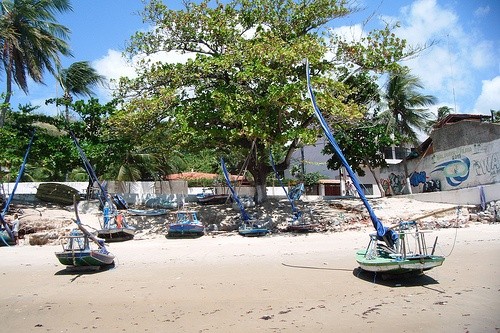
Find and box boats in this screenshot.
[0,57,462,278]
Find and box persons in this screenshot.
[5,213,19,244]
[104,196,117,224]
